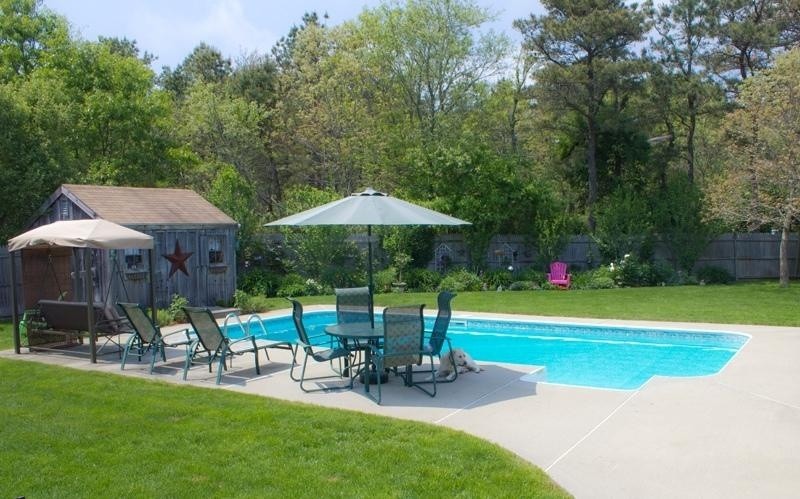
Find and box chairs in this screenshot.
[115,295,198,380]
[361,302,439,406]
[180,304,296,385]
[546,259,574,292]
[394,286,459,385]
[282,297,355,393]
[329,285,375,377]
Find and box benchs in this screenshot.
[41,299,130,361]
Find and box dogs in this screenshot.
[425,349,482,381]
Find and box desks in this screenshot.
[319,321,392,386]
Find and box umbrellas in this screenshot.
[8,214,156,366]
[264,188,474,329]
